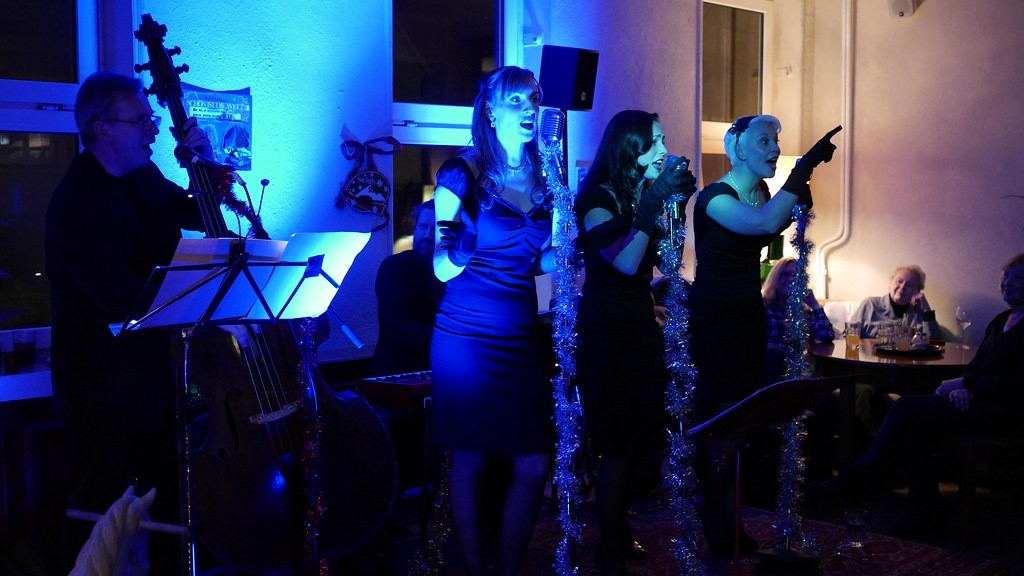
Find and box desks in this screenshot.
[811,337,980,530]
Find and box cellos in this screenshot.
[125,9,403,574]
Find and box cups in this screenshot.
[800,522,819,552]
[5,331,36,372]
[895,326,912,351]
[845,509,867,547]
[903,313,917,337]
[845,323,861,349]
[878,320,902,350]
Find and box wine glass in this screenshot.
[956,306,975,350]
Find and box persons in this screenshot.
[576,111,698,575]
[375,201,449,481]
[763,254,1024,531]
[694,113,842,575]
[43,73,219,576]
[430,67,585,575]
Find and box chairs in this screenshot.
[925,362,1024,553]
[739,342,857,514]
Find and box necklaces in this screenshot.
[502,158,527,170]
[728,173,761,206]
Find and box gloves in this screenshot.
[436,209,479,267]
[633,156,696,238]
[760,184,813,246]
[780,125,843,196]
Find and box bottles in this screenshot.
[912,330,930,351]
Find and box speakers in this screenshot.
[539,44,599,110]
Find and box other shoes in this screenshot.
[810,463,866,494]
[594,551,628,576]
[621,539,645,558]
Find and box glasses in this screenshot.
[93,114,162,133]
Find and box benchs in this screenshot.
[817,298,862,338]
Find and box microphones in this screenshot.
[539,109,568,186]
[663,155,687,230]
[175,145,246,185]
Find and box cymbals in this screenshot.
[358,367,434,386]
